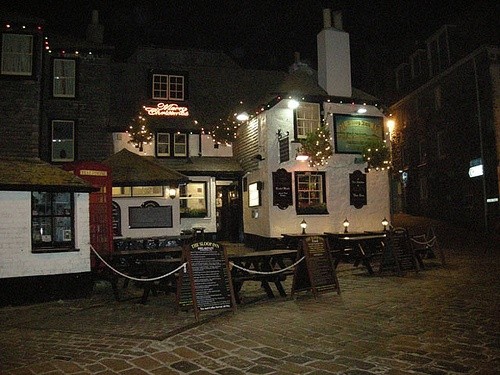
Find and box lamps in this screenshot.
[343,217,350,233]
[300,218,308,235]
[381,217,389,233]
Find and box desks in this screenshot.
[113,226,395,303]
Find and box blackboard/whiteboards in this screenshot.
[173,238,239,323]
[290,234,341,303]
[378,225,418,277]
